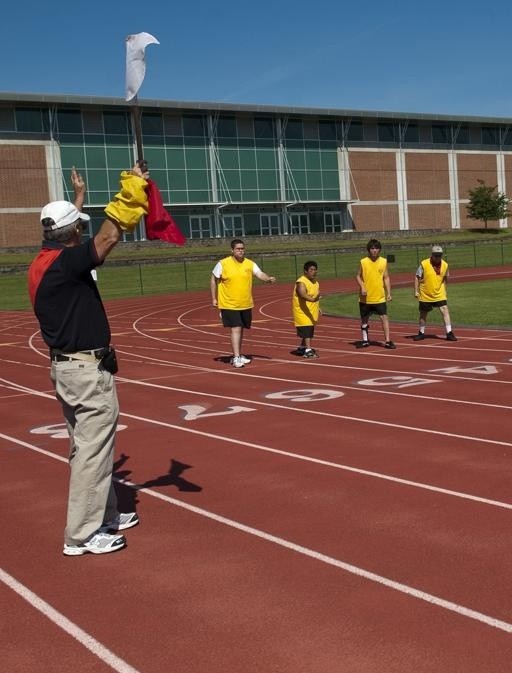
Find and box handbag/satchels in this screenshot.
[101,349,118,375]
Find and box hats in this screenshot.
[431,245,443,253]
[39,200,91,233]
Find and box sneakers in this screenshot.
[62,531,127,557]
[231,354,252,364]
[414,330,425,341]
[384,340,396,349]
[232,357,245,368]
[302,349,319,358]
[357,340,370,348]
[296,347,315,354]
[98,512,140,533]
[446,330,457,341]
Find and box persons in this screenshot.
[28,164,151,557]
[414,246,458,340]
[293,261,322,359]
[210,239,276,368]
[356,239,397,348]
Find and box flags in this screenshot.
[123,31,160,105]
[143,178,187,248]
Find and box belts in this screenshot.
[49,349,105,362]
